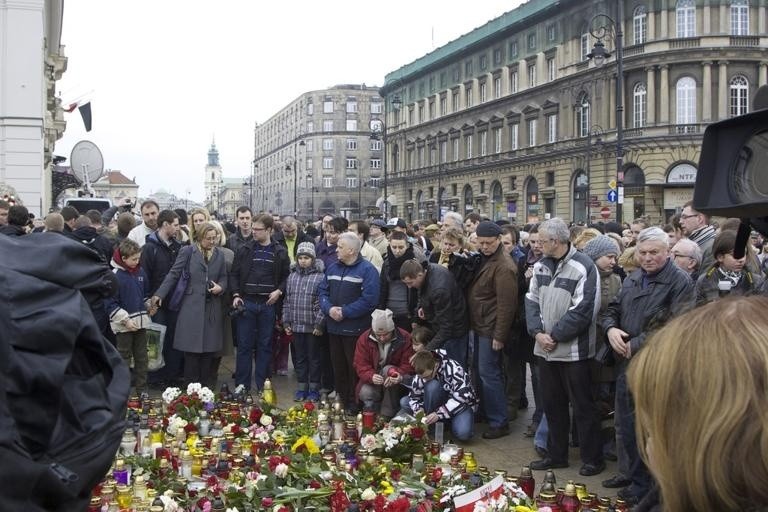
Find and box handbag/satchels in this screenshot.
[167,245,193,313]
[129,322,168,372]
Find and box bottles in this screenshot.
[86,377,628,512]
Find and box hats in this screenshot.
[476,221,504,238]
[582,235,620,262]
[369,219,386,228]
[369,307,396,335]
[294,241,317,262]
[380,218,406,232]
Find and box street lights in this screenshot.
[182,175,220,219]
[587,14,625,226]
[242,155,260,210]
[368,79,405,223]
[285,129,308,221]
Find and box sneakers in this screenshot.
[233,384,266,398]
[603,477,631,487]
[293,389,319,403]
[482,427,512,440]
[579,458,606,476]
[269,368,289,377]
[616,486,647,500]
[522,422,537,437]
[528,453,569,470]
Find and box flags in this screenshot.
[79,102,93,131]
[64,101,80,113]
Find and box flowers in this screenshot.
[144,381,551,511]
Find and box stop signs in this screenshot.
[600,206,611,221]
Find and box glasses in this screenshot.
[681,213,699,219]
[670,253,693,263]
